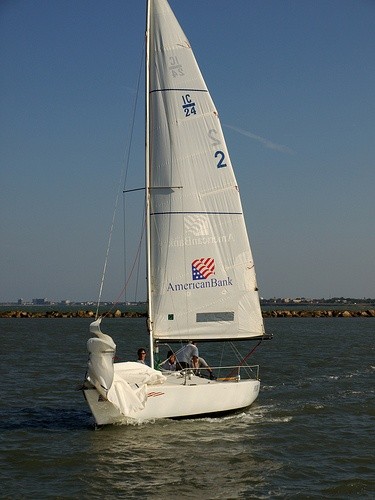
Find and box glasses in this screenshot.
[138,353,146,355]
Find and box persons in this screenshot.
[136,348,146,365]
[176,340,199,371]
[198,357,213,375]
[160,351,177,370]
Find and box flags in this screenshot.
[192,258,215,280]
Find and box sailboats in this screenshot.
[81,0,263,426]
